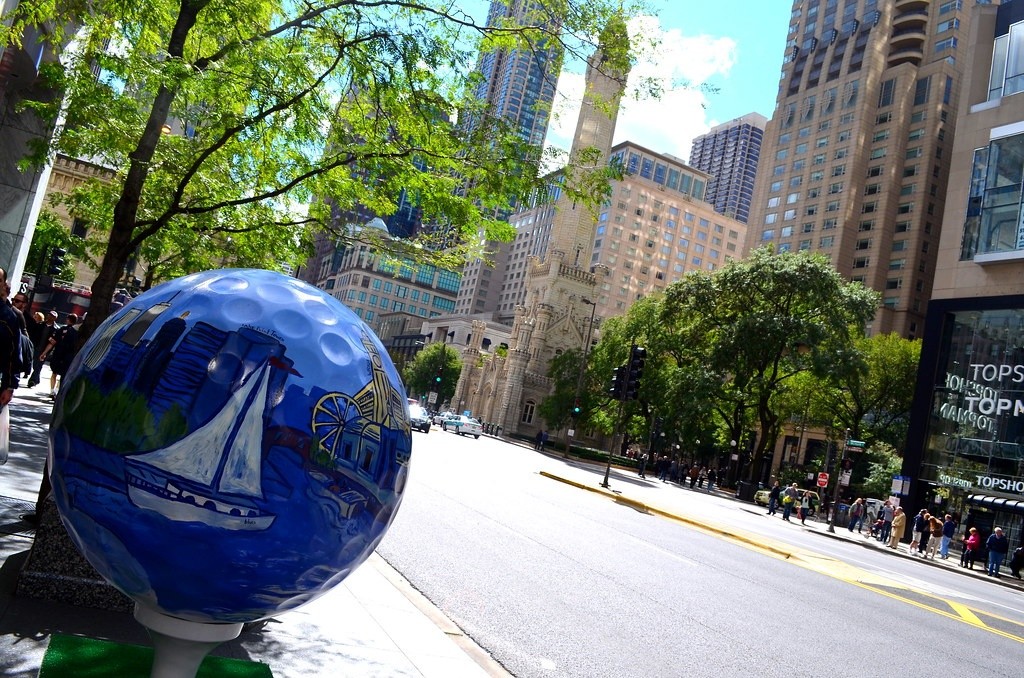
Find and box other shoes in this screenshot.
[886,543,897,549]
[879,539,887,543]
[907,550,934,561]
[996,575,1001,578]
[53,393,57,400]
[766,511,777,516]
[783,518,790,522]
[28,381,35,388]
[849,529,853,532]
[941,555,949,560]
[50,392,55,397]
[988,573,993,576]
[958,564,973,570]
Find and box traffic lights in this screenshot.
[574,398,581,413]
[609,365,626,400]
[655,419,664,436]
[47,244,67,276]
[621,344,648,403]
[839,458,850,469]
[431,365,444,392]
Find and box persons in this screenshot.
[848,497,868,533]
[879,501,906,549]
[1009,545,1024,580]
[958,527,979,570]
[797,490,812,524]
[478,417,482,424]
[534,429,548,452]
[0,267,79,412]
[987,526,1009,578]
[909,508,955,561]
[766,480,781,516]
[782,482,798,520]
[627,445,735,493]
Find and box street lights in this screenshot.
[563,299,597,458]
[726,439,737,489]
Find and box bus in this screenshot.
[22,272,123,324]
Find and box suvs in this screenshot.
[754,486,820,516]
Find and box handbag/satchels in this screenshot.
[0,403,10,465]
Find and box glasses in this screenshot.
[11,298,26,303]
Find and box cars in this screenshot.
[409,405,431,433]
[442,410,483,439]
[433,412,451,427]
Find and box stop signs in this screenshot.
[816,471,830,488]
